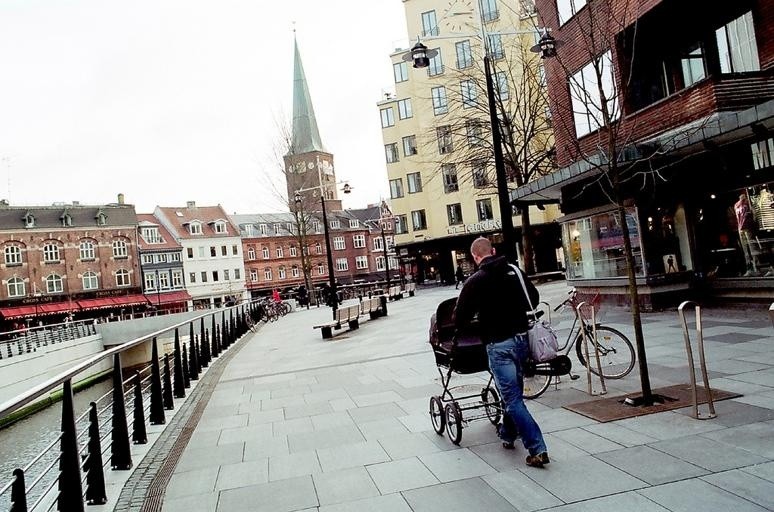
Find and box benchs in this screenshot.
[313,282,415,338]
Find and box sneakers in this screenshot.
[455,286,459,289]
[527,454,551,466]
[503,436,515,450]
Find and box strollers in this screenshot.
[430,297,512,443]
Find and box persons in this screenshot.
[269,276,347,314]
[4,314,82,343]
[751,188,774,276]
[732,194,760,278]
[451,234,554,467]
[664,255,678,272]
[451,264,466,290]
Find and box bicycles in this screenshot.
[258,298,292,322]
[244,312,258,333]
[522,288,637,399]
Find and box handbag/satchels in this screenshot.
[529,316,557,363]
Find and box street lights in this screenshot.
[402,28,566,266]
[293,155,350,329]
[363,208,401,293]
